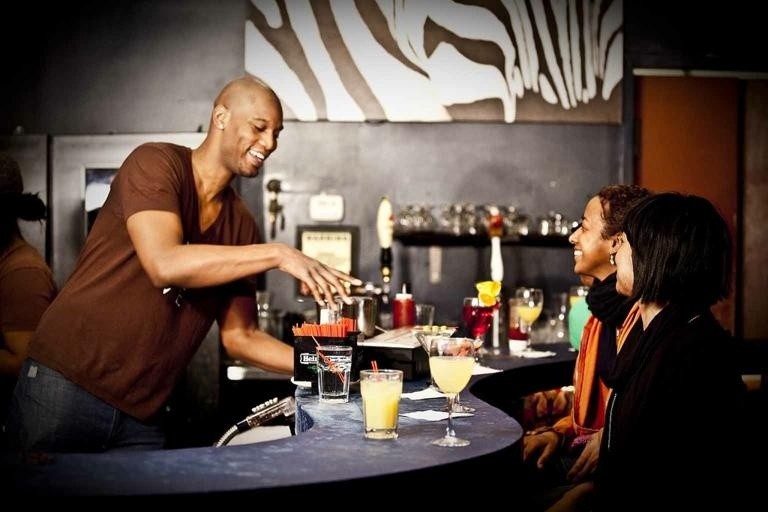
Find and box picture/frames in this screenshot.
[295,224,361,299]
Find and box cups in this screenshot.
[414,303,435,326]
[359,369,407,440]
[314,345,352,404]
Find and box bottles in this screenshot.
[378,282,418,331]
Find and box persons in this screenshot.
[4,76,363,456]
[536,188,750,510]
[0,151,60,385]
[521,183,652,485]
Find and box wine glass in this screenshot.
[409,323,485,413]
[427,355,475,447]
[461,295,495,356]
[514,286,545,355]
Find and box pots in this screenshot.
[297,295,377,337]
[258,302,287,340]
